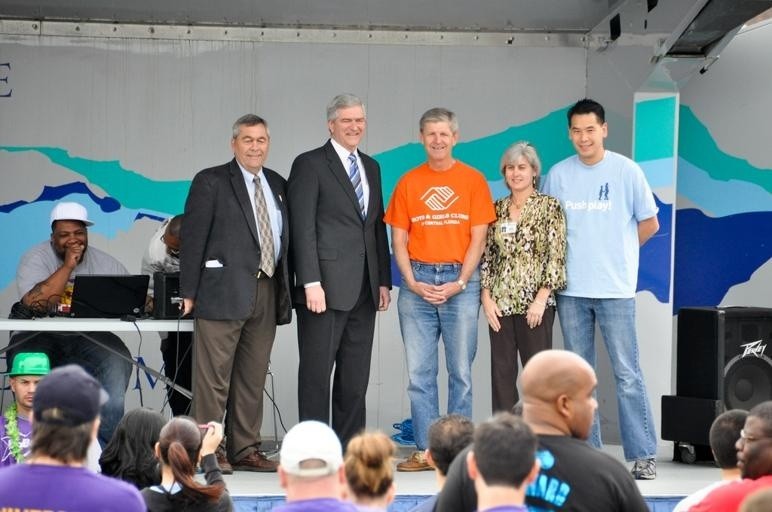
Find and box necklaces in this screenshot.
[510,197,527,209]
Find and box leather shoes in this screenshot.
[397,451,436,470]
[216,450,279,473]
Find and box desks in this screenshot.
[1,315,244,473]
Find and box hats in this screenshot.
[280,420,343,476]
[50,202,95,227]
[6,353,109,427]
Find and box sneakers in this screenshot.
[632,458,656,479]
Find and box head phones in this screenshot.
[46,291,68,318]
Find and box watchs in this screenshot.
[457,278,466,290]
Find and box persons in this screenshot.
[381,108,492,474]
[141,214,195,417]
[0,351,234,512]
[271,420,396,511]
[673,400,772,512]
[424,349,648,512]
[481,139,567,414]
[287,94,390,462]
[541,100,659,478]
[5,201,135,448]
[179,113,298,472]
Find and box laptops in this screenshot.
[69,274,150,318]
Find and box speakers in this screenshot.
[676,306,772,412]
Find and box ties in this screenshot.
[348,152,366,221]
[253,175,276,277]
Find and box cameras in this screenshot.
[198,424,211,436]
